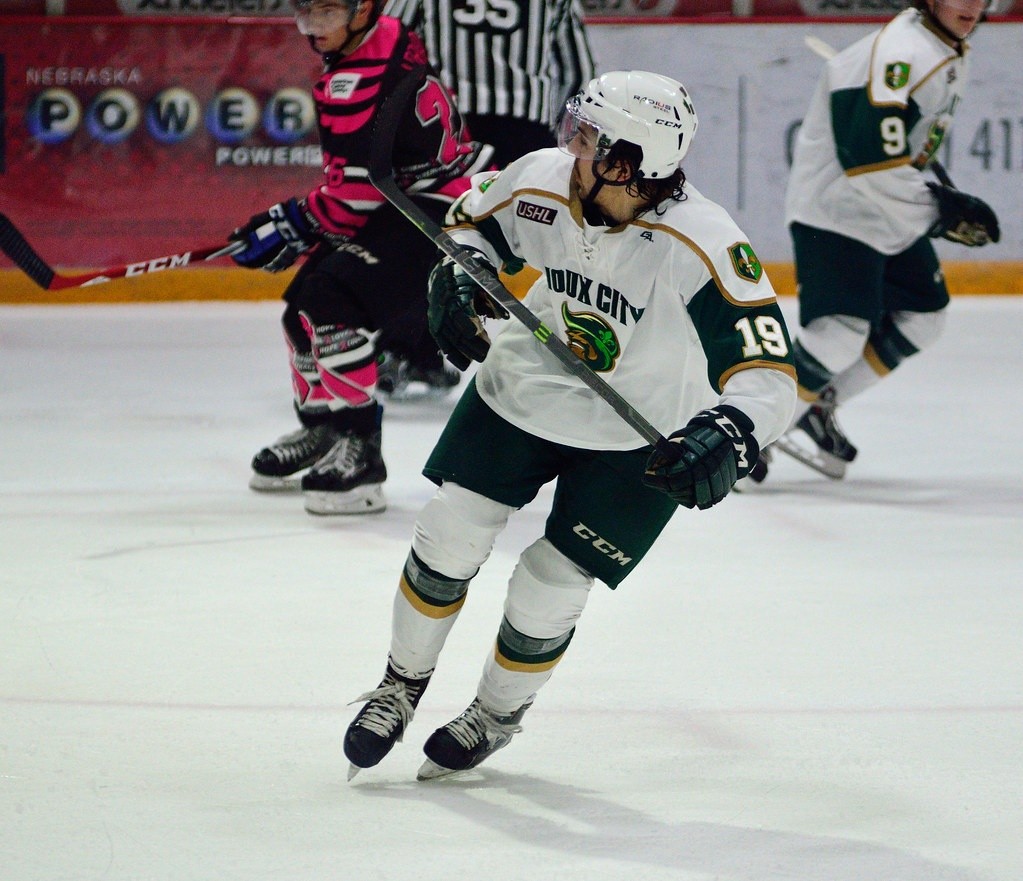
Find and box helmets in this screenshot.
[567,70,699,180]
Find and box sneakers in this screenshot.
[301,405,388,514]
[344,651,435,782]
[771,385,857,479]
[246,399,342,490]
[376,348,414,401]
[403,356,461,399]
[416,696,538,781]
[732,450,768,494]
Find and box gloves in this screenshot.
[259,201,323,274]
[926,180,1000,246]
[640,403,760,510]
[426,244,509,373]
[227,196,307,270]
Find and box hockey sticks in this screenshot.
[363,63,665,442]
[800,32,962,190]
[0,209,247,294]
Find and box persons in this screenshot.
[341,68,800,783]
[228,0,490,515]
[374,0,599,396]
[762,0,1014,479]
